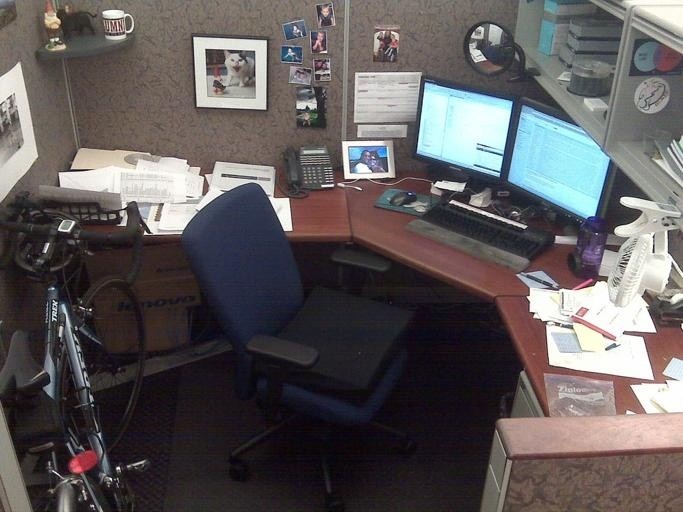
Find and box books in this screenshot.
[651,133,683,188]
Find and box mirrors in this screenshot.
[463,19,540,86]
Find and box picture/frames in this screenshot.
[0,63,40,211]
[188,31,270,112]
[339,138,397,181]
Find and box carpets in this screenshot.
[60,363,174,512]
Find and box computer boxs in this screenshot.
[510,369,545,418]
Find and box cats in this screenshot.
[221,49,256,88]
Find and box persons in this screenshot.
[283,4,334,126]
[2,118,20,152]
[44,1,64,49]
[351,150,372,174]
[368,151,385,173]
[377,29,398,60]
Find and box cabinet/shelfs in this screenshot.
[515,0,632,174]
[602,1,683,221]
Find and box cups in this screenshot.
[101,9,134,42]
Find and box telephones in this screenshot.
[283,144,335,189]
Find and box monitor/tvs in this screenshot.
[413,74,520,190]
[502,95,618,227]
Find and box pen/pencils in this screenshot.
[572,279,593,290]
[521,272,559,289]
[650,399,668,413]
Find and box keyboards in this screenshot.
[405,202,556,273]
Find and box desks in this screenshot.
[499,288,682,418]
[12,161,352,247]
[349,165,618,296]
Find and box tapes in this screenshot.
[669,293,683,305]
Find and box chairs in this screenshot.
[178,181,421,507]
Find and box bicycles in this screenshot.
[1,192,156,512]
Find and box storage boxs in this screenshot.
[94,300,193,351]
[81,252,204,316]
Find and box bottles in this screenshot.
[569,216,610,282]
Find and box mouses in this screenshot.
[389,190,417,207]
